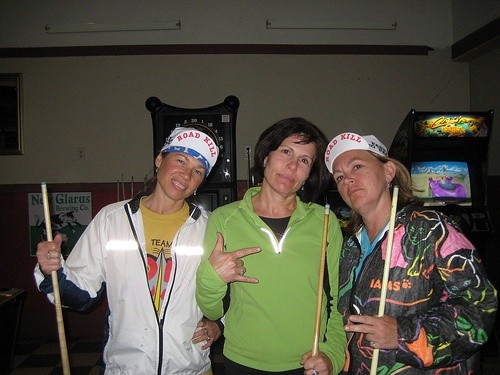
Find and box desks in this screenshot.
[0,286,28,375]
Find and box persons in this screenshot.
[322,130,500,375]
[196,117,347,375]
[33,121,231,375]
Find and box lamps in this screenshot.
[266,18,397,31]
[45,18,182,35]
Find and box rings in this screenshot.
[204,329,208,335]
[205,334,210,340]
[313,367,318,375]
[240,268,244,276]
[372,342,375,349]
[47,251,50,259]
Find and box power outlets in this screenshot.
[244,144,251,155]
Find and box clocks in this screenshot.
[144,95,241,212]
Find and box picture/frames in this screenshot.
[0,73,24,156]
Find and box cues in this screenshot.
[311,204,331,352]
[367,186,399,372]
[41,177,70,375]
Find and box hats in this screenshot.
[325,132,388,175]
[160,127,219,177]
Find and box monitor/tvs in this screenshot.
[411,161,472,207]
